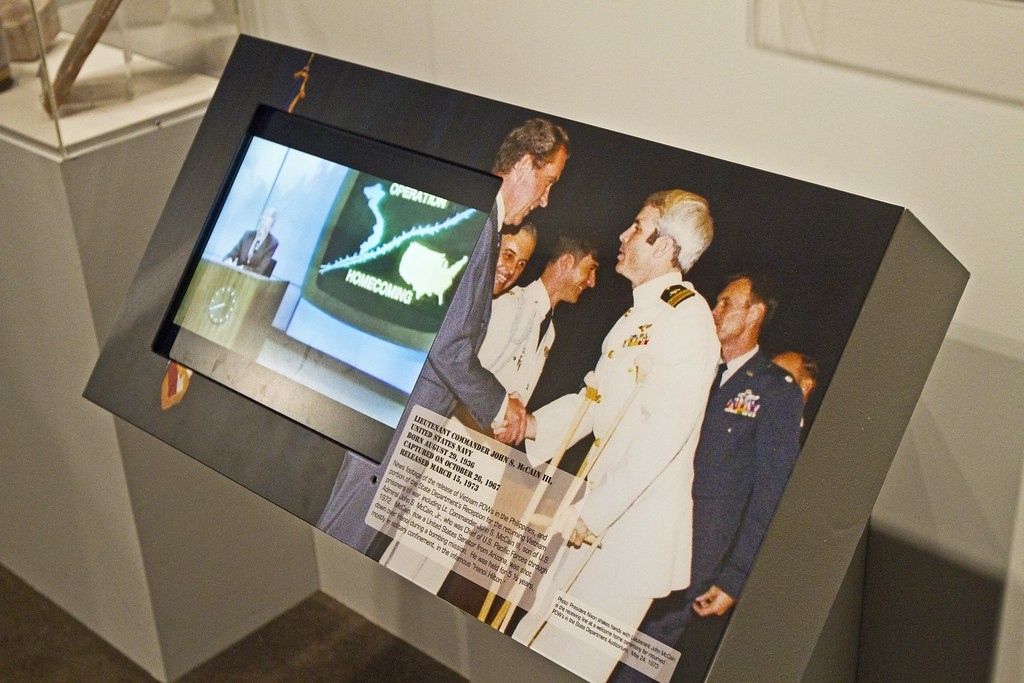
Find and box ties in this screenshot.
[536,308,553,352]
[495,224,503,261]
[707,362,728,405]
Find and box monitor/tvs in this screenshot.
[151,105,503,467]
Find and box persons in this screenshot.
[316,117,823,683]
[221,207,278,275]
